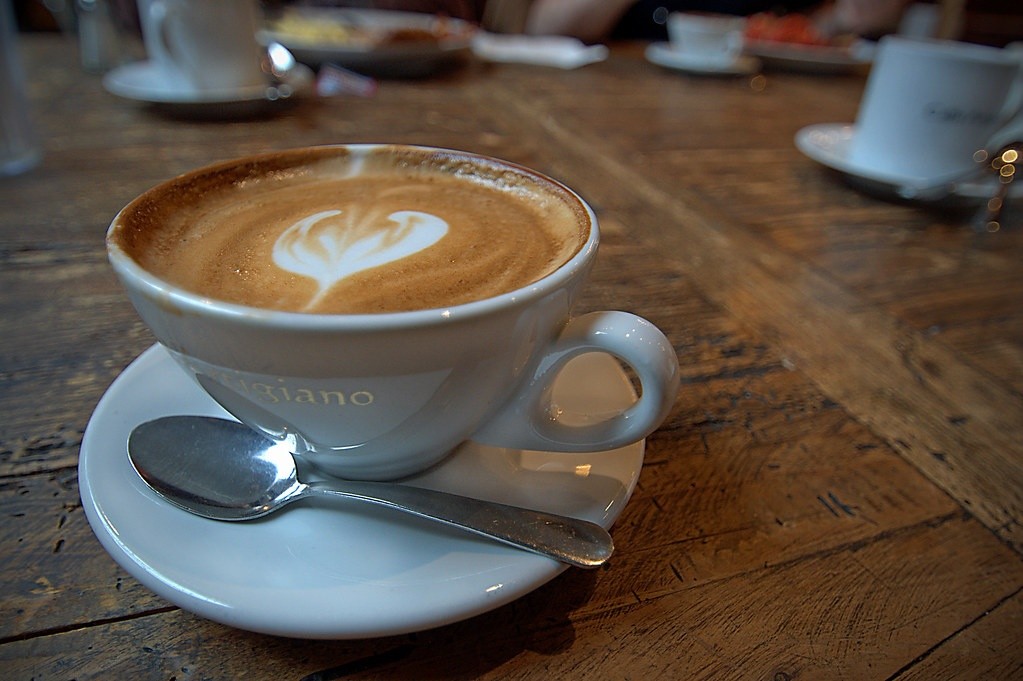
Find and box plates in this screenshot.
[77,340,646,640]
[100,60,310,105]
[748,39,878,71]
[258,6,480,70]
[645,43,762,77]
[793,121,1023,200]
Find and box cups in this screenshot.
[106,142,684,485]
[136,0,269,89]
[671,14,747,61]
[850,32,1023,184]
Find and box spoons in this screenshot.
[972,145,1023,231]
[266,41,294,101]
[124,415,616,570]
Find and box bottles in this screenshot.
[0,0,47,181]
[74,0,131,76]
